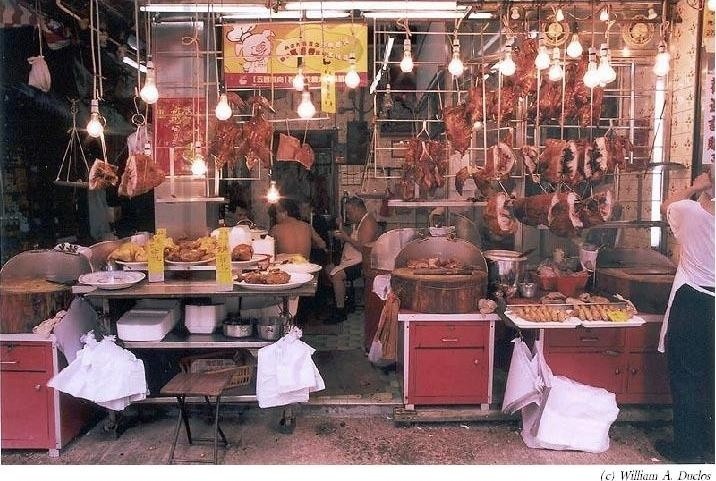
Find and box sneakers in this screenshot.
[322,310,347,325]
[655,439,707,464]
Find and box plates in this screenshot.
[163,258,216,266]
[230,254,269,266]
[78,269,146,290]
[115,297,182,343]
[115,259,149,267]
[503,310,649,329]
[210,294,240,328]
[182,304,218,335]
[233,261,323,292]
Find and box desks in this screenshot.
[71,271,319,439]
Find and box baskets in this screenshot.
[180,349,256,393]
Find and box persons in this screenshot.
[654,164,715,462]
[222,197,378,324]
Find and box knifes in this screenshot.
[45,276,74,286]
[623,268,677,276]
[414,267,474,276]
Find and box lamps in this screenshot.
[548,0,563,80]
[86,0,102,138]
[139,0,158,103]
[582,0,616,88]
[500,0,515,76]
[399,0,413,72]
[320,0,333,83]
[567,0,582,57]
[215,0,231,120]
[292,0,315,119]
[447,0,464,75]
[191,0,205,175]
[382,20,393,111]
[267,0,279,202]
[344,8,360,88]
[534,0,550,69]
[652,0,670,75]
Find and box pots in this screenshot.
[482,250,527,285]
[223,320,281,341]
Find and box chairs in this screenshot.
[345,221,387,312]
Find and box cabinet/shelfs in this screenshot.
[0,333,96,457]
[397,308,502,410]
[504,310,673,404]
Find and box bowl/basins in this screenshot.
[519,283,537,299]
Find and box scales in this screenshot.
[355,124,394,199]
[52,94,90,189]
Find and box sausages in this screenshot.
[516,304,618,323]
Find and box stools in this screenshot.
[159,371,232,464]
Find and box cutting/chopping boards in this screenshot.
[596,267,677,315]
[391,266,489,315]
[1,274,74,334]
